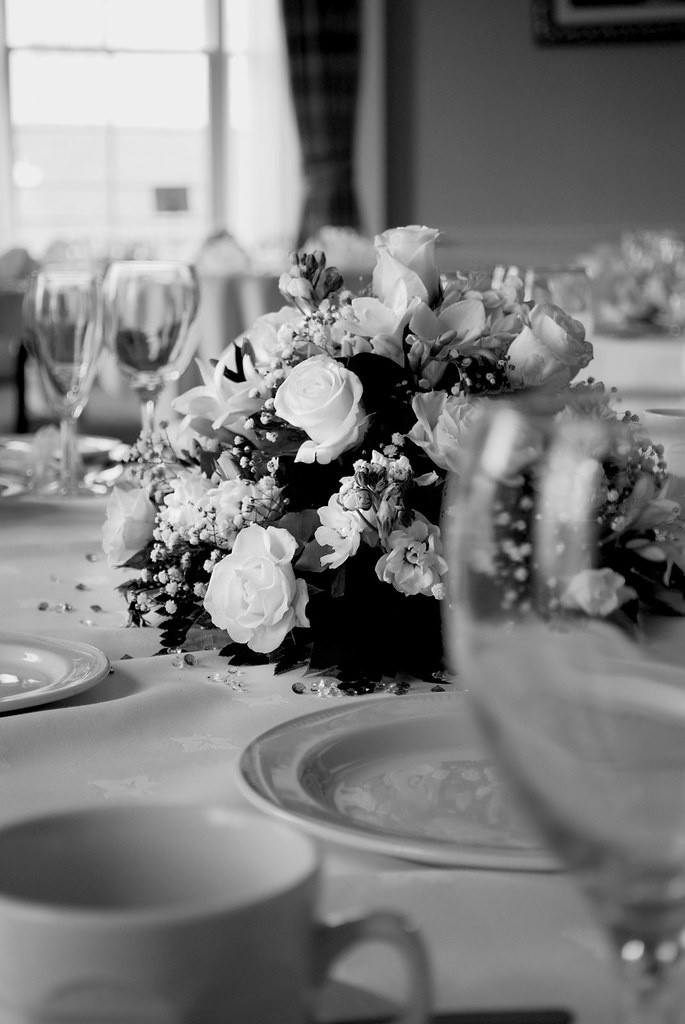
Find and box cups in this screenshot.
[0,804,430,1022]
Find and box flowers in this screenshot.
[94,212,685,687]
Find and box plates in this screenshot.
[234,687,684,872]
[0,446,48,498]
[0,628,110,713]
[8,434,120,456]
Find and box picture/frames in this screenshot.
[529,0,685,48]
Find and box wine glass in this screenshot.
[98,262,199,489]
[22,269,105,496]
[441,394,682,1023]
